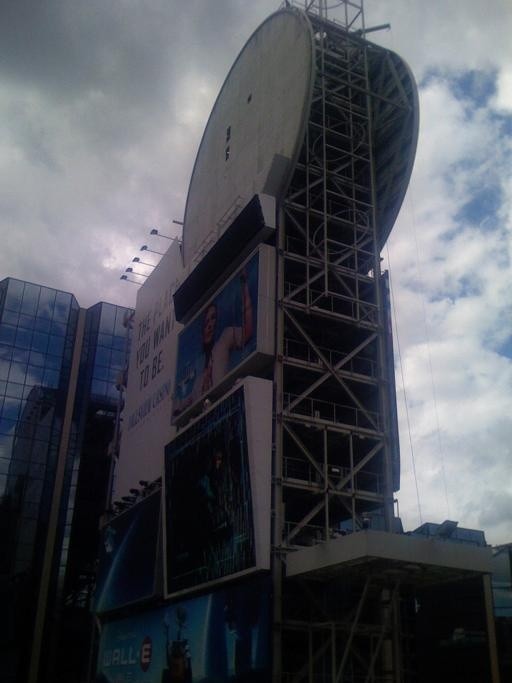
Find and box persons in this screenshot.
[169,265,253,418]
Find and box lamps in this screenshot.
[105,479,155,517]
[116,228,176,286]
